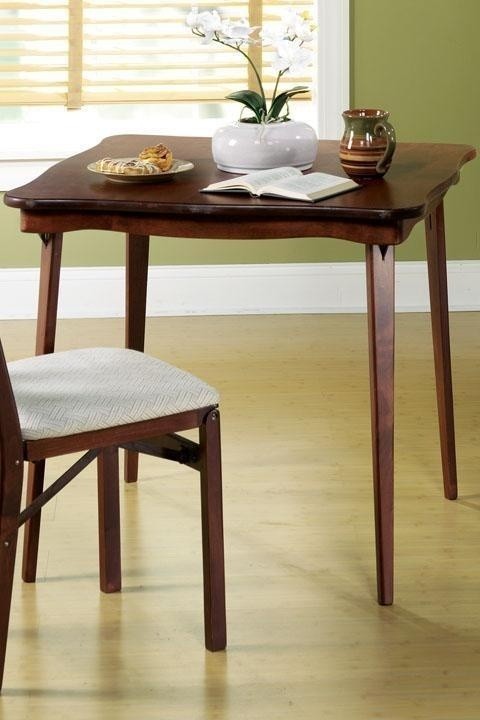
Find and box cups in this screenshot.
[338,108,397,179]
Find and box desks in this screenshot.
[4,132,477,608]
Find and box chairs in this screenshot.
[0,311,228,698]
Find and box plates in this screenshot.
[86,157,195,183]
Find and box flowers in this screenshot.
[183,7,321,125]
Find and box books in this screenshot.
[198,166,362,203]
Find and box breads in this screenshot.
[92,143,172,174]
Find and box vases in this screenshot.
[212,117,319,174]
